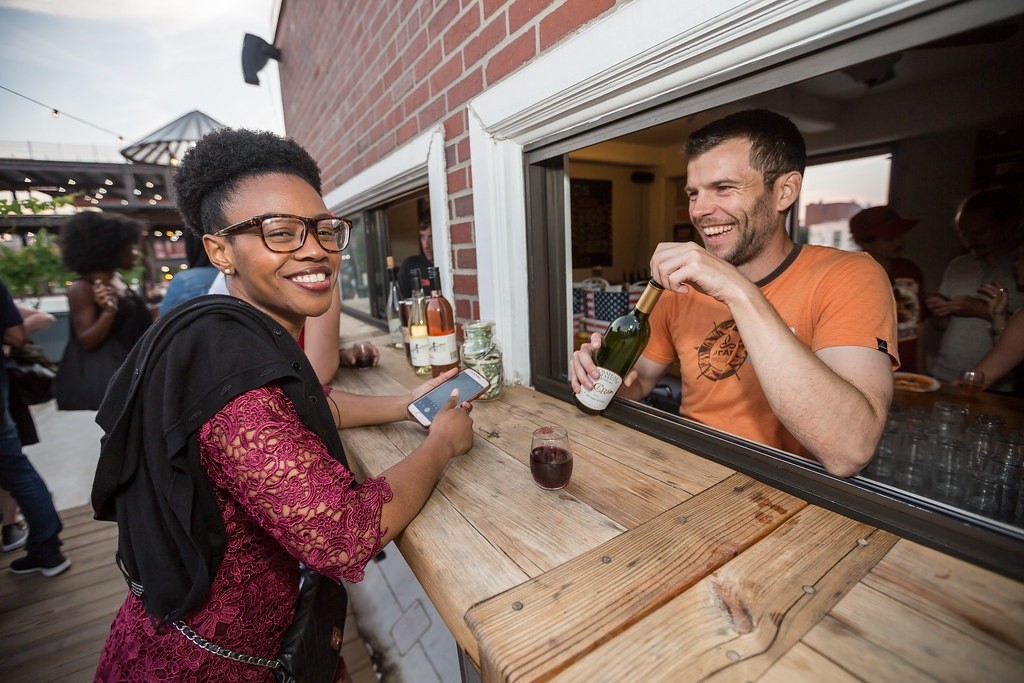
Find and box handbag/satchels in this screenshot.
[273,566,348,683]
[55,277,129,415]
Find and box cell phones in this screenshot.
[95,278,119,312]
[406,367,491,429]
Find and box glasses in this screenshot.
[213,213,353,253]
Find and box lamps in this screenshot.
[242,32,284,86]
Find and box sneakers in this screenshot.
[0,517,28,551]
[9,549,71,577]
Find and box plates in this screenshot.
[893,372,940,392]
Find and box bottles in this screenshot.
[572,279,665,415]
[407,268,432,376]
[424,266,459,379]
[459,320,504,403]
[385,255,405,348]
[574,316,591,353]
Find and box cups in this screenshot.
[861,399,1024,528]
[398,295,431,367]
[529,426,574,490]
[957,367,985,396]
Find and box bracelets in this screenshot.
[990,328,1005,337]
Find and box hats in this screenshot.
[851,205,919,242]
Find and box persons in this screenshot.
[926,182,1024,397]
[160,227,218,314]
[89,128,474,683]
[0,281,71,578]
[396,207,434,298]
[570,109,901,479]
[211,267,379,386]
[850,205,929,377]
[60,210,156,349]
